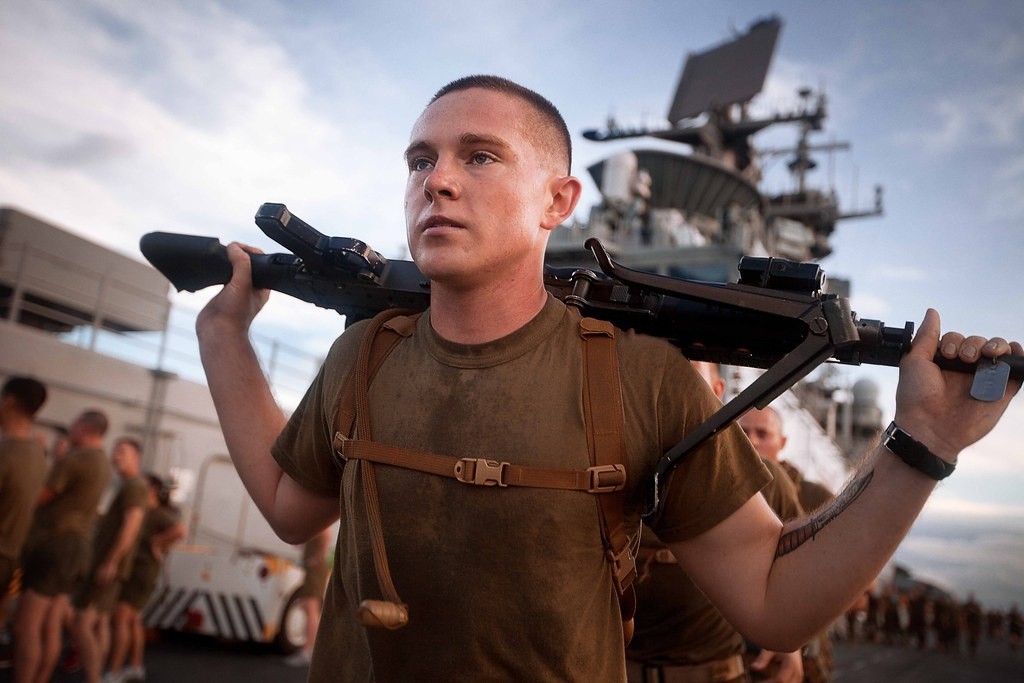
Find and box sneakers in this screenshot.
[123,666,146,681]
[104,669,128,683]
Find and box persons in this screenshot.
[193,71,1024,683]
[1,375,182,683]
[620,358,805,683]
[826,584,1023,662]
[734,408,840,682]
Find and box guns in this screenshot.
[137,201,1022,506]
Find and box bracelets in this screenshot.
[880,420,957,481]
